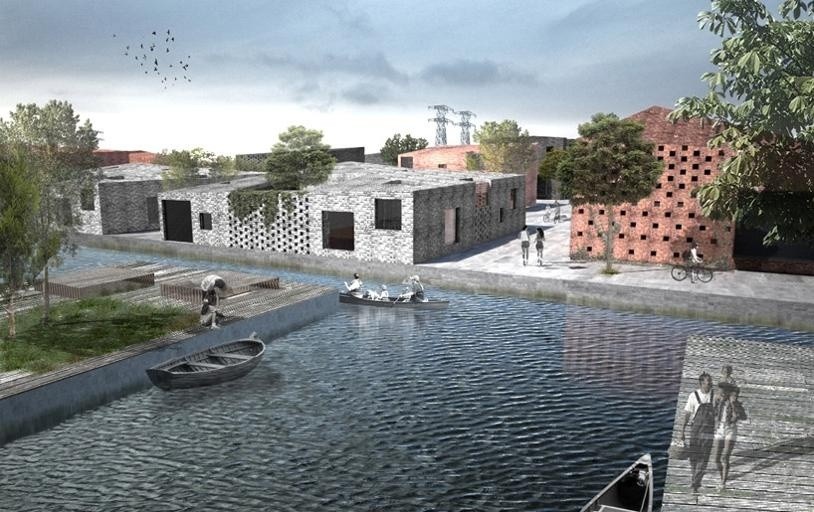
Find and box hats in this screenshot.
[410,275,420,282]
[379,284,387,290]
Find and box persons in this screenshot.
[392,285,412,304]
[374,284,389,300]
[517,225,531,266]
[401,274,425,303]
[531,226,545,268]
[687,243,704,285]
[342,272,362,302]
[363,285,380,301]
[543,199,561,225]
[197,274,236,307]
[199,287,224,330]
[678,362,748,495]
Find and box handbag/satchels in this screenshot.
[668,439,692,461]
